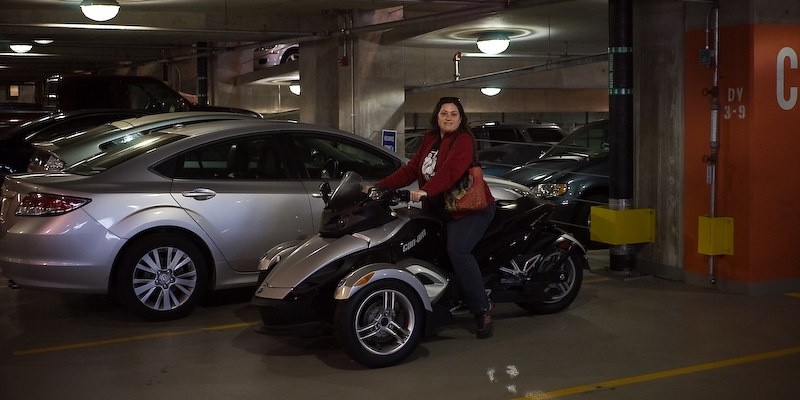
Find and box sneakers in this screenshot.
[476,301,494,340]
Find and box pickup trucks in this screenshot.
[0,73,196,177]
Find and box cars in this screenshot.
[0,118,532,325]
[502,119,611,251]
[399,123,568,181]
[25,110,281,173]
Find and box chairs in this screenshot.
[229,143,261,172]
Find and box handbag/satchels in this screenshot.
[444,130,487,210]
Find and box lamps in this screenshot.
[80,0,121,22]
[477,33,511,56]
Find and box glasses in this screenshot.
[440,96,461,102]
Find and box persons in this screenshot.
[364,96,497,336]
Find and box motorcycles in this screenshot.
[246,166,588,365]
